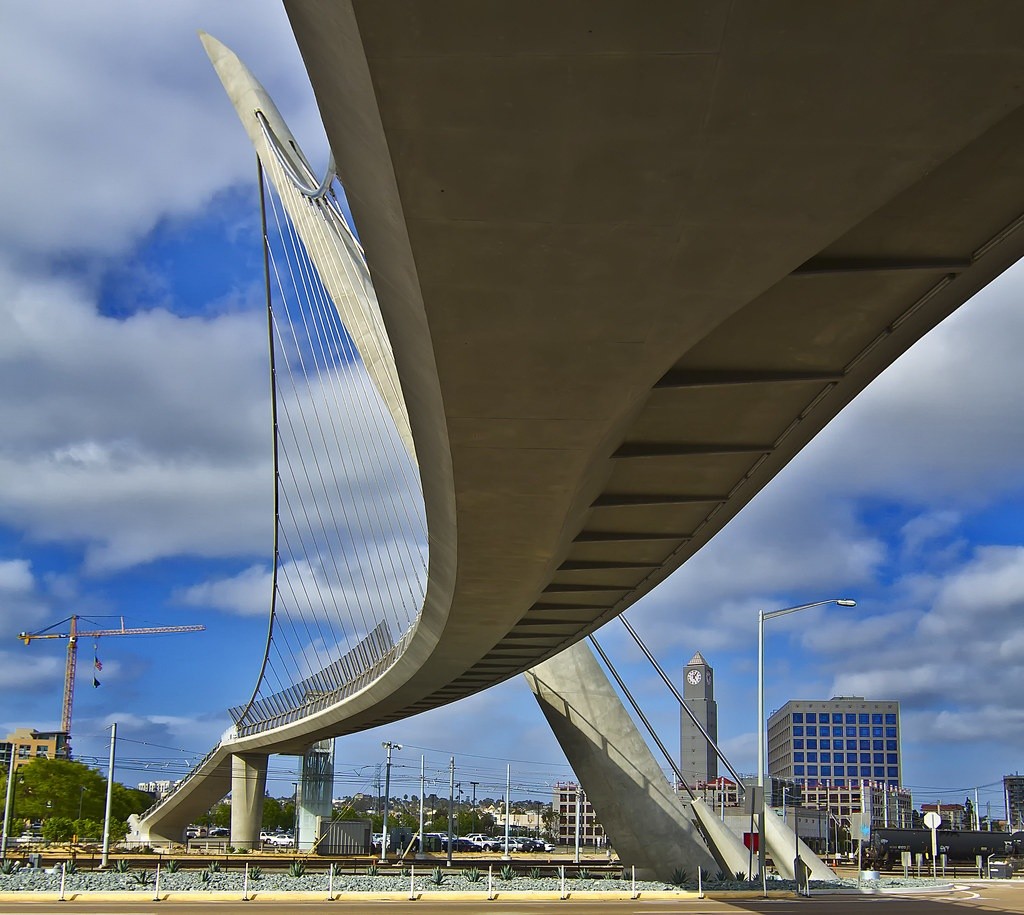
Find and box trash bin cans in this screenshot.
[990,863,1013,879]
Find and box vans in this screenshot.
[185,826,230,838]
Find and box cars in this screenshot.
[260,830,295,848]
[372,833,391,845]
[423,830,556,854]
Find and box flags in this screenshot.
[93,677,101,688]
[94,656,102,671]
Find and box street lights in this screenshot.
[756,599,856,884]
[291,783,297,834]
[469,781,480,834]
[782,786,790,823]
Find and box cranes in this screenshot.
[16,613,205,736]
